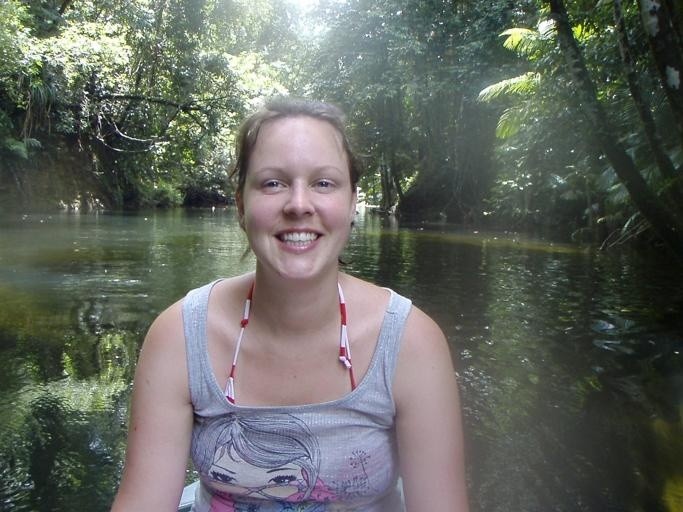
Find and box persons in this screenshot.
[108,95,470,512]
